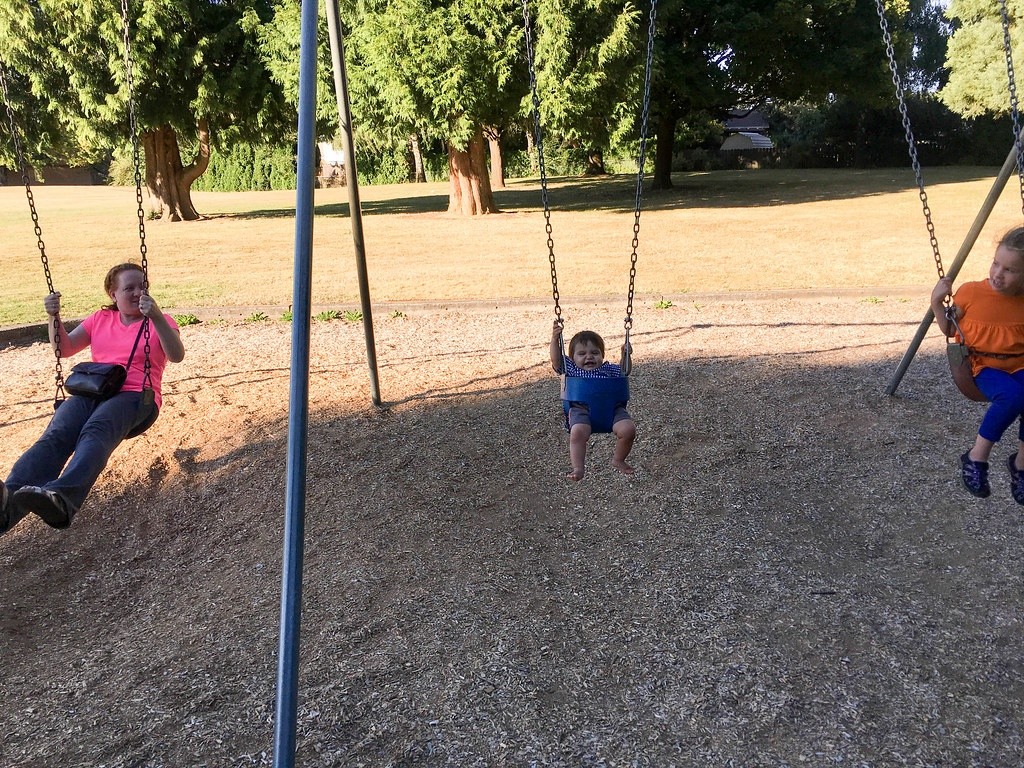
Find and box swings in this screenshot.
[519,0,657,435]
[1,0,158,443]
[874,0,1024,404]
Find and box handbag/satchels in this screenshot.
[64,361,126,399]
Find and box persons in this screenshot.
[931,227,1024,507]
[549,318,637,481]
[0,262,185,537]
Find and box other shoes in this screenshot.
[0,479,10,536]
[13,485,73,530]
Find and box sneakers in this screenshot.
[1006,453,1024,505]
[957,447,991,498]
